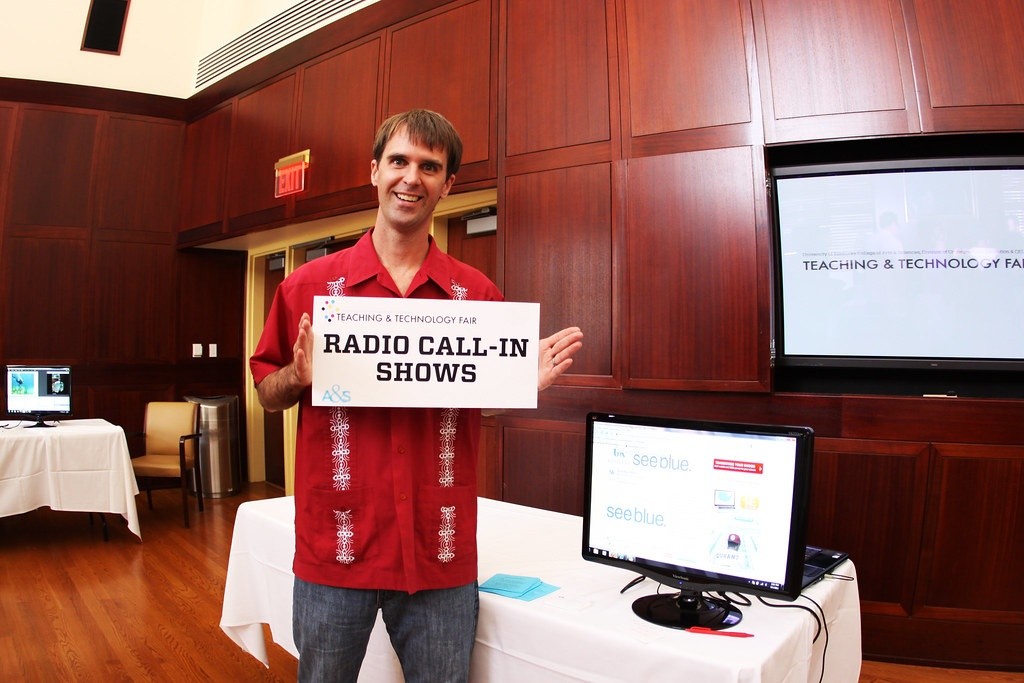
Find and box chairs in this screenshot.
[119,402,204,528]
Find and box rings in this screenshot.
[552,359,555,365]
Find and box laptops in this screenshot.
[802,546,849,590]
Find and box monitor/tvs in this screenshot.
[4,365,73,428]
[770,156,1024,396]
[582,411,815,630]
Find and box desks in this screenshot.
[0,418,143,544]
[219,496,862,683]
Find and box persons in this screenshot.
[250,110,583,683]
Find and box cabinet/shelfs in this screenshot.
[0,0,1024,673]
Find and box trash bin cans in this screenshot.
[179,392,245,498]
